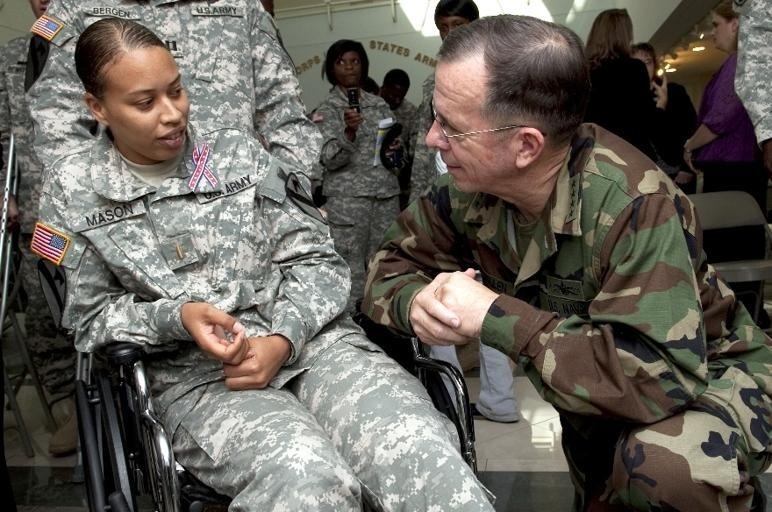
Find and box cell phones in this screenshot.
[347,88,360,113]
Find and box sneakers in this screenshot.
[41,393,78,454]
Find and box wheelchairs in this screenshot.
[72,297,478,512]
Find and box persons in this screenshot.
[408,1,480,196]
[585,1,772,334]
[308,39,406,325]
[34,17,499,512]
[378,69,415,153]
[0,0,115,455]
[23,1,325,215]
[364,13,771,512]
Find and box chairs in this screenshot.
[0,251,59,457]
[684,191,772,335]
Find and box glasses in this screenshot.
[432,100,528,143]
